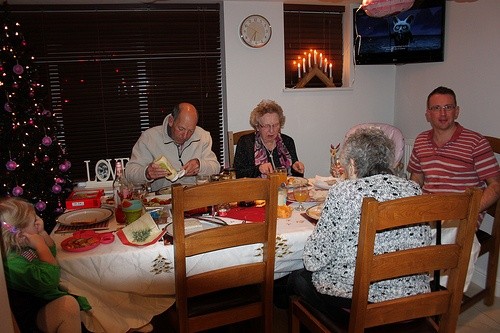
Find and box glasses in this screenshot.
[429,104,456,111]
[257,121,280,129]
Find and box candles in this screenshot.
[314,50,316,64]
[308,53,311,67]
[303,58,307,73]
[319,53,323,68]
[324,57,327,73]
[297,63,300,78]
[330,63,333,78]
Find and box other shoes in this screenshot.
[273,287,297,310]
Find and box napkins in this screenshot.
[116,213,167,247]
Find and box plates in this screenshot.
[55,207,114,226]
[314,174,338,188]
[60,233,101,252]
[305,203,322,221]
[158,184,195,195]
[164,216,227,238]
[286,188,329,203]
[287,176,309,188]
[150,193,173,210]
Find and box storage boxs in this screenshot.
[66,189,104,210]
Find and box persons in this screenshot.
[273,127,431,333]
[0,198,92,333]
[406,86,500,231]
[232,98,304,179]
[124,101,220,192]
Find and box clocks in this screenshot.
[240,15,274,48]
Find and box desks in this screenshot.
[50,176,482,333]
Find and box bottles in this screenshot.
[155,154,179,183]
[113,162,128,225]
[211,167,253,216]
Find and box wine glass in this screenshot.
[292,177,309,212]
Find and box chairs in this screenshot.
[171,173,279,333]
[227,129,255,168]
[289,186,483,333]
[460,137,500,312]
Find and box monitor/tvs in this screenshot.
[353,0,445,65]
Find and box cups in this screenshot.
[266,166,287,191]
[122,200,142,225]
[196,174,209,186]
[130,181,147,201]
